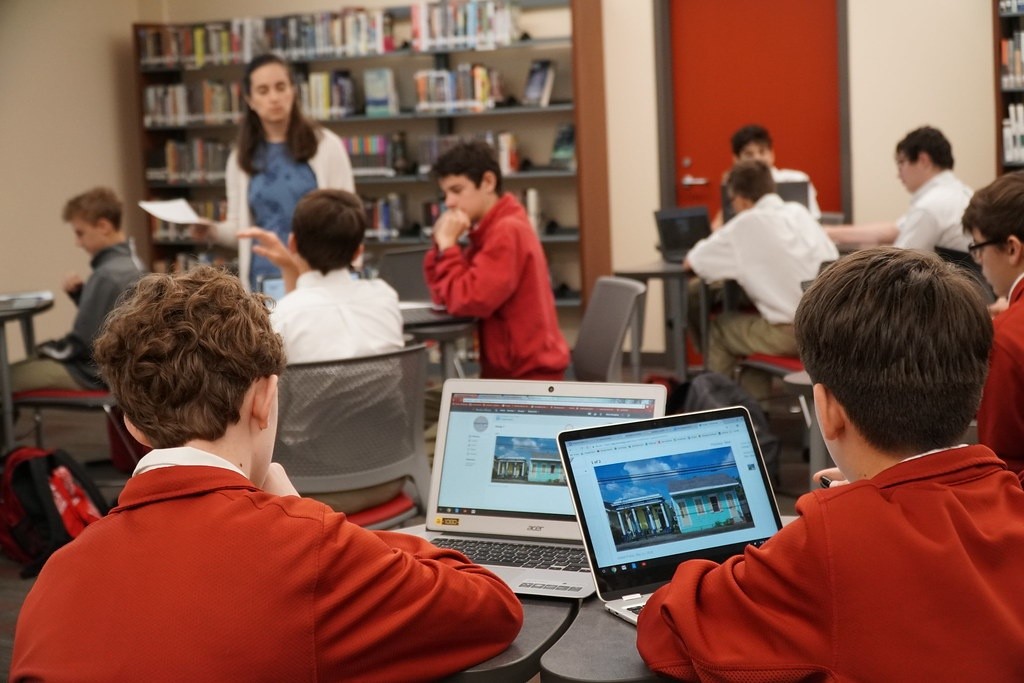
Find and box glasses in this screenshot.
[968,232,1024,260]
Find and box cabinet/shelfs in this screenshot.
[993,0,1024,177]
[131,0,615,317]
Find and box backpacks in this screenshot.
[0,446,109,578]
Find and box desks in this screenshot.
[0,292,54,449]
[388,522,657,683]
[399,301,481,379]
[782,368,827,489]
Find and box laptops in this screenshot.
[257,270,362,309]
[720,180,810,225]
[557,406,785,626]
[420,377,667,598]
[655,206,712,263]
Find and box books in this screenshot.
[414,65,505,116]
[527,187,544,234]
[363,193,409,242]
[416,130,519,175]
[423,198,445,239]
[166,136,228,186]
[520,60,558,107]
[550,122,578,172]
[152,253,222,274]
[1001,30,1024,165]
[151,198,227,243]
[202,78,247,125]
[139,6,412,68]
[144,83,193,127]
[363,68,398,117]
[343,131,407,176]
[420,0,521,52]
[299,70,356,120]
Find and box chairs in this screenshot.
[573,273,647,385]
[272,342,431,532]
[729,352,820,423]
[14,388,141,465]
[615,263,697,386]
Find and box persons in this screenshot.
[711,126,975,251]
[962,172,1024,482]
[0,185,149,454]
[681,158,841,397]
[637,248,1024,683]
[237,186,409,514]
[37,306,524,682]
[423,139,571,380]
[190,56,363,312]
